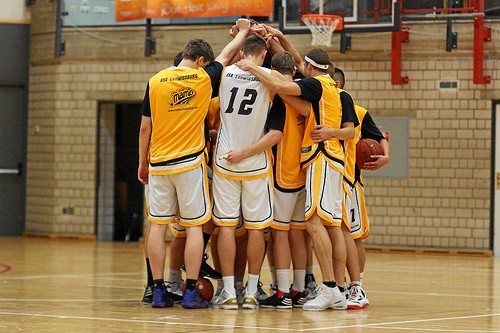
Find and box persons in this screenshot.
[137,18,390,311]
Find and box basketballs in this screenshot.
[356,138,384,169]
[182,277,214,303]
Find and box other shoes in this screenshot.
[210,283,224,304]
[168,290,184,304]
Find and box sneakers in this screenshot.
[241,287,260,309]
[258,286,292,309]
[181,285,209,308]
[302,283,348,311]
[270,284,278,294]
[289,284,307,308]
[181,252,224,280]
[210,287,238,310]
[235,281,246,304]
[245,280,269,301]
[345,284,370,309]
[305,281,322,298]
[151,286,174,308]
[142,284,152,305]
